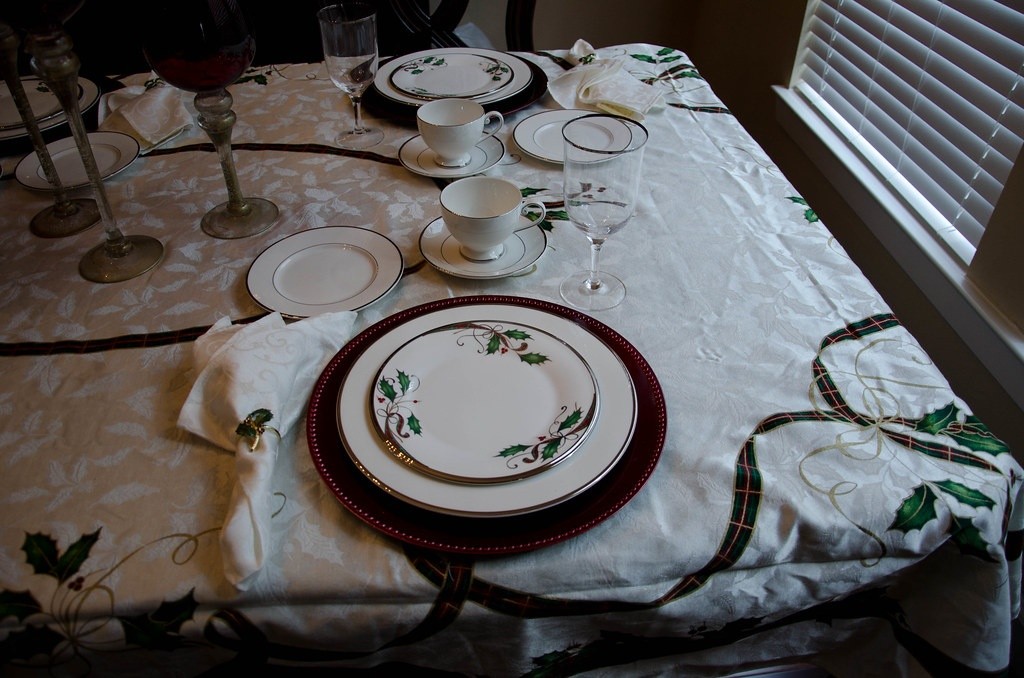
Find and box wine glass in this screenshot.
[1,22,100,238]
[559,113,649,312]
[317,3,385,151]
[12,0,163,283]
[133,0,279,238]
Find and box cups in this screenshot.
[416,99,503,167]
[439,177,546,261]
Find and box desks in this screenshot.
[0,44,1024,678]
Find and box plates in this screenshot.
[512,109,633,166]
[0,75,100,141]
[417,215,548,279]
[369,320,602,482]
[244,225,405,320]
[359,54,549,129]
[397,131,506,177]
[335,305,638,517]
[389,52,514,99]
[0,78,85,129]
[307,293,667,557]
[14,132,139,191]
[373,46,532,105]
